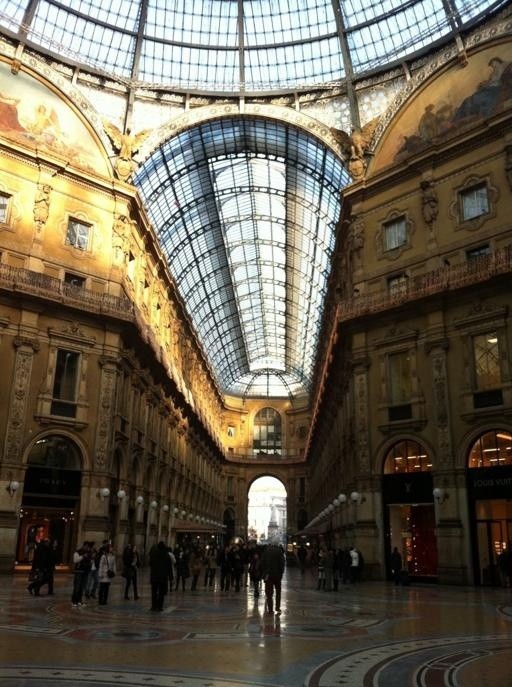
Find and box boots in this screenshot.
[267,596,281,614]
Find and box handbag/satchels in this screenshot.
[190,564,201,576]
[106,569,115,579]
[28,571,44,582]
[121,564,138,581]
[206,568,215,577]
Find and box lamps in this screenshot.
[100,486,221,528]
[431,484,450,505]
[6,480,20,497]
[318,491,360,519]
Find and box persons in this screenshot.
[391,546,402,580]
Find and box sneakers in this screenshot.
[123,594,140,601]
[149,605,163,612]
[72,593,109,607]
[168,584,240,592]
[316,587,338,592]
[24,587,56,597]
